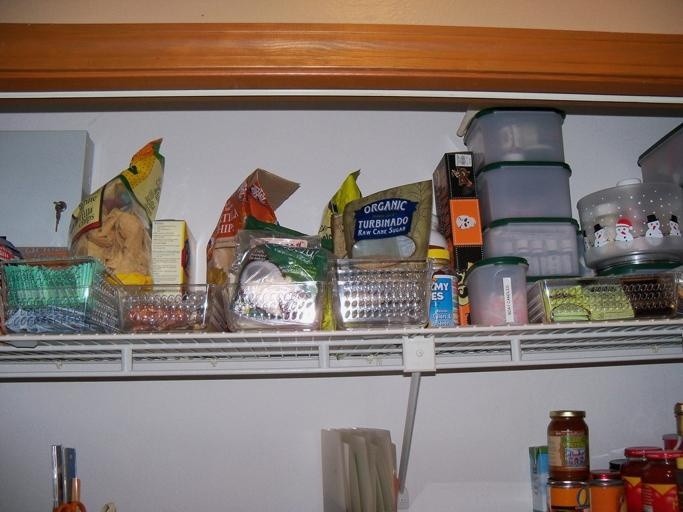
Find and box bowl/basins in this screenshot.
[576,177,683,271]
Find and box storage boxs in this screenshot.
[1,104,682,341]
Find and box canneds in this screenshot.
[545,403,683,512]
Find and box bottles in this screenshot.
[424,247,460,326]
